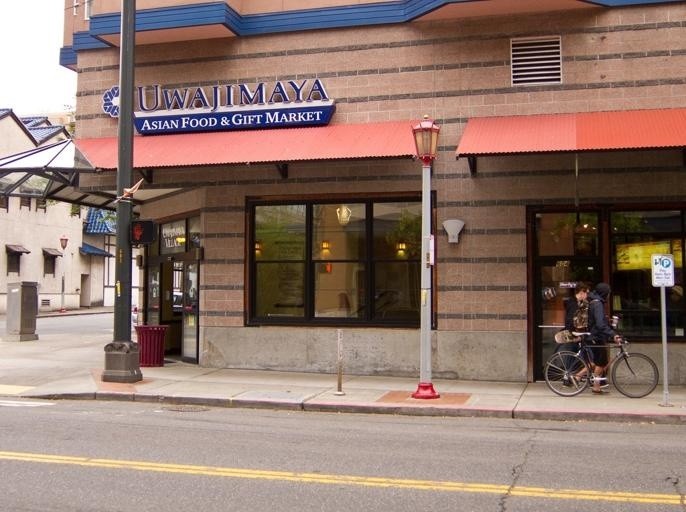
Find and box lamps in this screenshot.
[336,204,352,225]
[395,240,408,253]
[254,240,263,254]
[322,239,329,249]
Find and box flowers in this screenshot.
[133,307,139,326]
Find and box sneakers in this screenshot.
[562,375,610,394]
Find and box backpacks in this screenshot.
[572,299,597,329]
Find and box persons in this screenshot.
[567,282,624,396]
[562,280,610,390]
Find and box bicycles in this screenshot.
[544,332,659,398]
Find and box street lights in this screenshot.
[58,234,69,314]
[411,115,441,399]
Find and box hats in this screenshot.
[595,282,612,300]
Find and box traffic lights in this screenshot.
[130,218,157,245]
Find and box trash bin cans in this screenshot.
[134,325,169,366]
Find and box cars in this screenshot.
[132,291,182,327]
